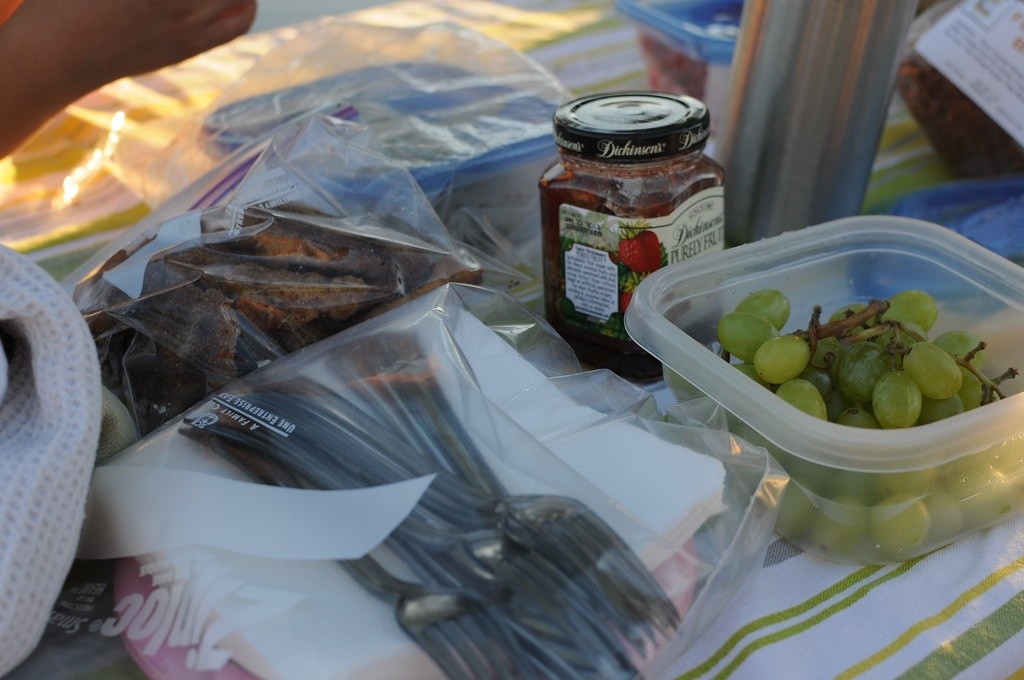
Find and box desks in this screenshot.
[0,0,1024,680]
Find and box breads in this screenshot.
[73,200,484,437]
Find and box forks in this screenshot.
[178,332,681,680]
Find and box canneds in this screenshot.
[536,92,727,382]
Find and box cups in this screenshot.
[715,0,918,246]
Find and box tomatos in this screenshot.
[641,35,706,102]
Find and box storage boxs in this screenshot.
[201,57,554,258]
[625,208,1024,560]
[618,0,746,131]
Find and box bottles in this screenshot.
[539,90,725,383]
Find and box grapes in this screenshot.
[684,288,1024,556]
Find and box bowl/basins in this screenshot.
[615,0,745,133]
[624,215,1024,566]
[200,59,564,256]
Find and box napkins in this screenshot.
[115,307,727,680]
